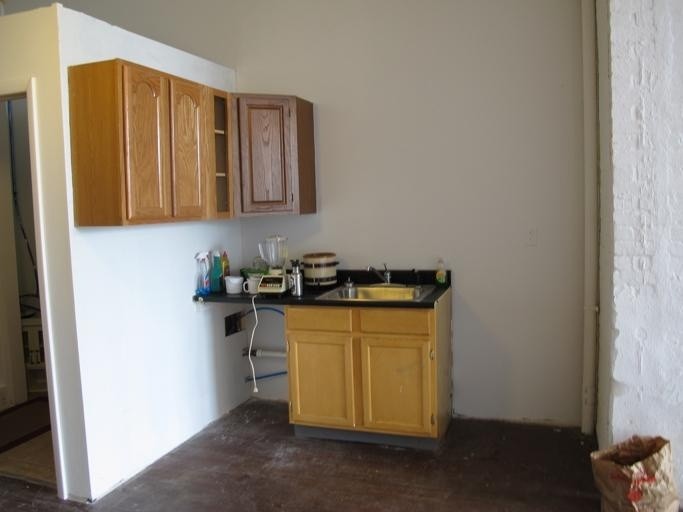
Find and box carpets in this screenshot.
[0,396,50,457]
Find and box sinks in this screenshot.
[315,284,437,303]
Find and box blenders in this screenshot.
[256,235,291,294]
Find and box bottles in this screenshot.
[339,277,357,299]
[289,264,302,297]
[210,249,230,294]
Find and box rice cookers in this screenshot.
[302,252,338,288]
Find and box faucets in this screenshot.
[366,261,392,284]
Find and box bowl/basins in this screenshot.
[238,267,265,281]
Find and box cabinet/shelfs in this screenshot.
[283,289,452,454]
[205,84,233,220]
[229,90,316,215]
[67,58,205,226]
[20,317,47,393]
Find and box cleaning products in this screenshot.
[432,255,448,290]
[210,250,224,292]
[222,250,231,276]
[195,251,211,297]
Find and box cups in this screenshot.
[242,279,259,294]
[225,276,243,296]
